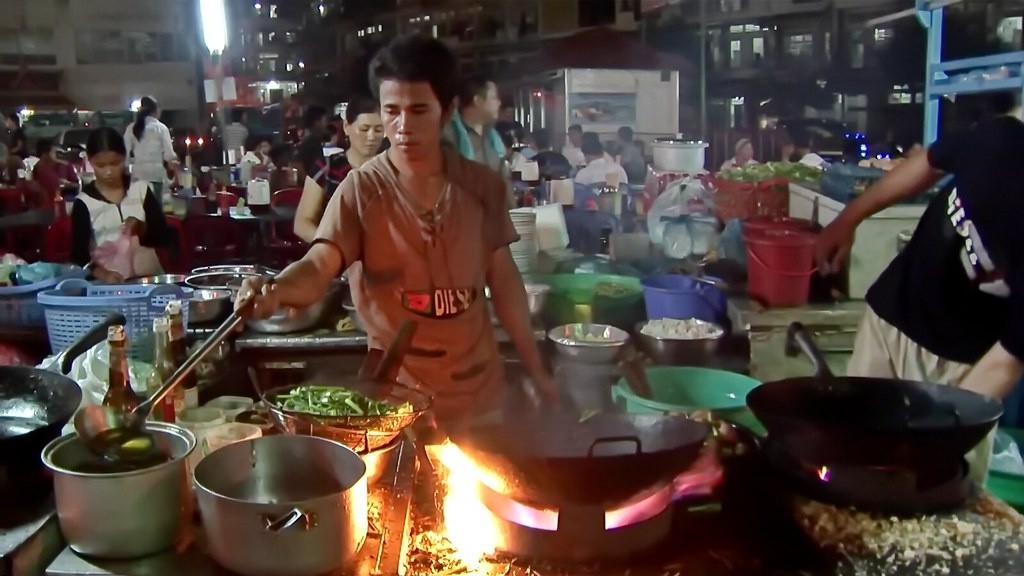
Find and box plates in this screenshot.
[507,207,538,285]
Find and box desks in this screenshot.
[230,315,556,398]
[199,211,262,263]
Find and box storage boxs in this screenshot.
[818,196,929,301]
[701,178,787,223]
[788,184,819,221]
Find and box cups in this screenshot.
[997,14,1023,52]
[206,422,263,453]
[204,395,255,420]
[174,405,226,475]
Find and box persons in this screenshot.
[0,87,390,284]
[813,90,1024,495]
[441,74,648,216]
[234,34,560,428]
[720,133,827,181]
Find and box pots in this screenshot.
[444,322,1003,506]
[0,314,369,575]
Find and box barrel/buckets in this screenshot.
[739,215,821,308]
[639,273,724,324]
[608,364,767,433]
[247,176,271,212]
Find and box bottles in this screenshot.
[100,323,140,414]
[163,300,200,409]
[145,317,187,426]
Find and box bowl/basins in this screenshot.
[119,260,366,334]
[361,436,403,489]
[483,273,644,364]
[985,425,1024,508]
[634,319,725,365]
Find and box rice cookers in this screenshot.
[649,132,710,173]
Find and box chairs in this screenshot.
[0,156,312,266]
[513,169,637,255]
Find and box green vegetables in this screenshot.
[715,162,823,190]
[276,384,392,414]
